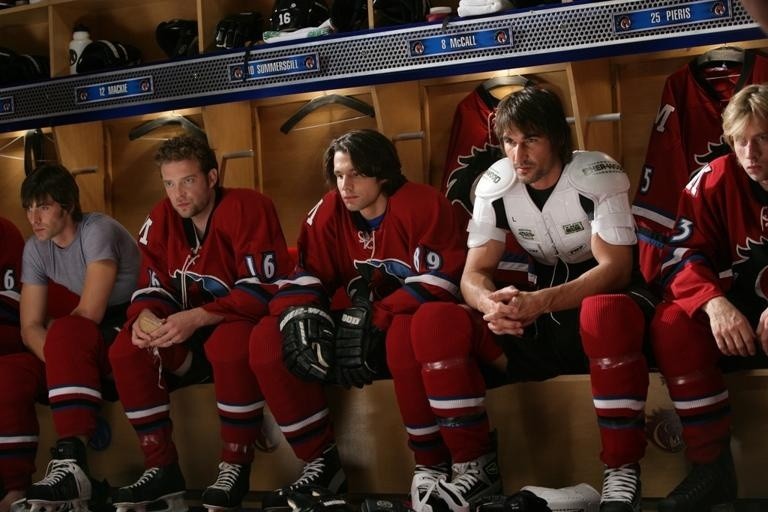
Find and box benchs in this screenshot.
[24,367,768,510]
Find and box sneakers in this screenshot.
[657,436,738,512]
[202,463,249,512]
[0,489,26,512]
[505,483,602,512]
[410,460,452,512]
[112,463,189,512]
[421,427,505,512]
[27,437,98,512]
[599,461,641,512]
[278,439,347,512]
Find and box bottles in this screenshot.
[67,31,92,75]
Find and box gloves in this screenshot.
[215,11,262,48]
[280,305,376,390]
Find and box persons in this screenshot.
[0,217,25,354]
[650,84,768,512]
[0,166,140,504]
[409,84,657,512]
[248,128,468,512]
[106,131,295,508]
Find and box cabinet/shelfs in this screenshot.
[0,1,767,286]
